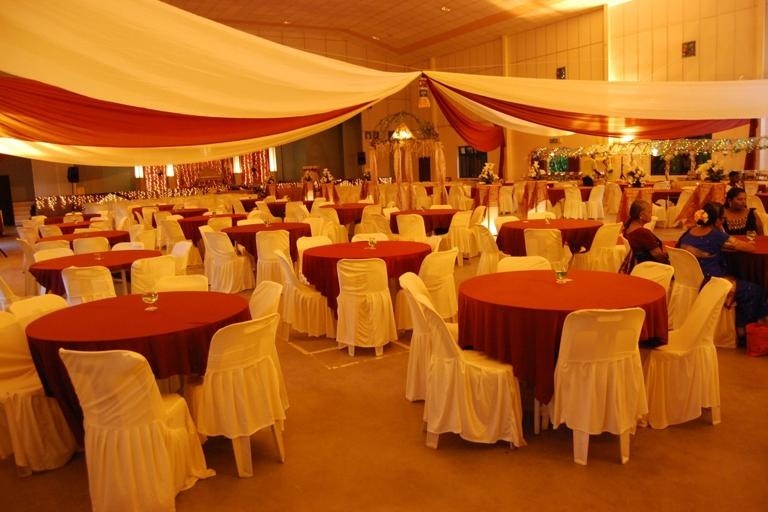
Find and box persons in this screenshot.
[626,171,642,188]
[622,199,675,281]
[720,187,768,237]
[725,171,743,193]
[674,201,768,350]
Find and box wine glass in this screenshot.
[368,236,376,250]
[141,286,158,311]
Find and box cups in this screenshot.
[94,253,103,260]
[544,215,552,225]
[262,216,271,225]
[745,229,756,241]
[551,260,568,284]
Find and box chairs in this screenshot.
[1,170,767,512]
